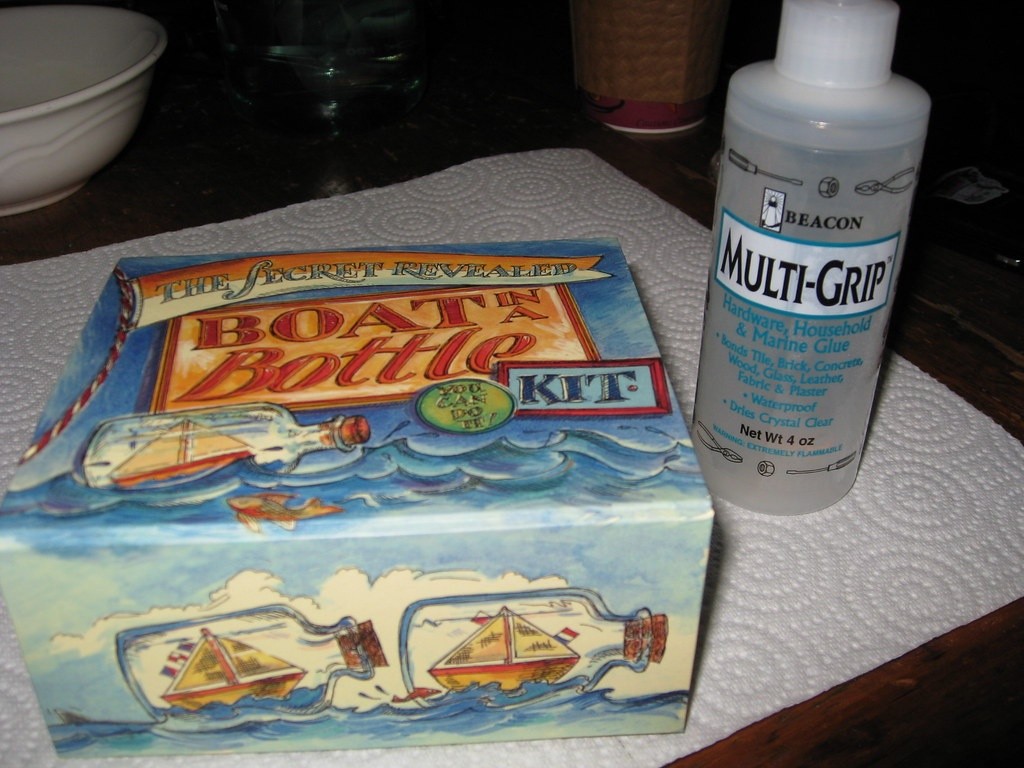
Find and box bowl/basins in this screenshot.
[0,6,169,216]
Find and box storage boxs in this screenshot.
[0,236,709,760]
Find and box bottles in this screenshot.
[689,0,930,517]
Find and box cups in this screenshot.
[569,0,730,135]
[214,0,427,134]
